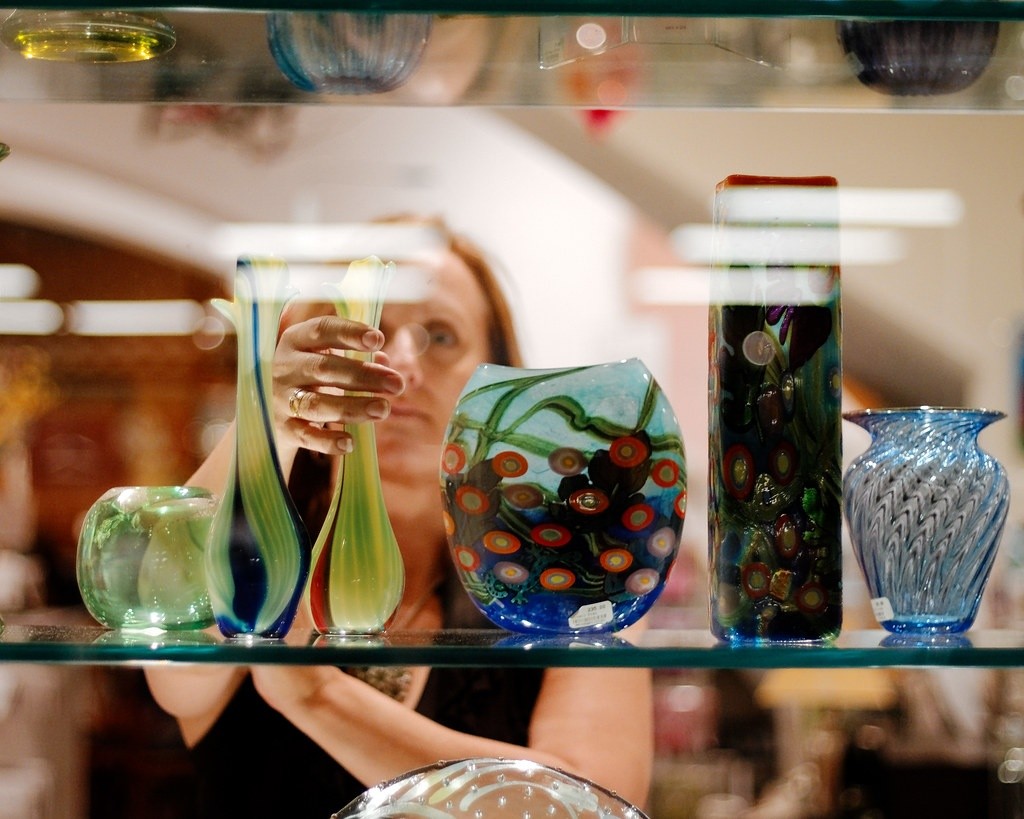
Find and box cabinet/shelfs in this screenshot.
[0,0,1023,667]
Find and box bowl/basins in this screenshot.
[76,487,219,632]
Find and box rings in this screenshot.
[288,388,307,415]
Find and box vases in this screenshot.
[76,482,216,644]
[709,170,841,644]
[307,259,406,650]
[844,405,1007,639]
[202,256,307,641]
[443,358,686,645]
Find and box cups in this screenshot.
[834,19,1000,95]
[265,9,432,94]
[1,8,176,64]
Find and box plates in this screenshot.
[439,353,688,640]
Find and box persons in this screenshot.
[82,216,654,819]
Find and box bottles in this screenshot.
[840,407,1011,638]
[205,239,311,642]
[307,249,406,635]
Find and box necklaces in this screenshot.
[344,574,441,704]
